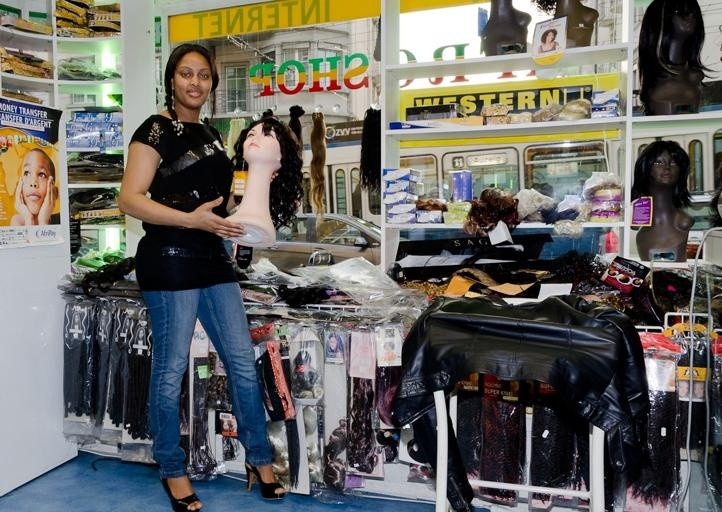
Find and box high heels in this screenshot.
[244,458,287,500]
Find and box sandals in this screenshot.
[159,475,201,512]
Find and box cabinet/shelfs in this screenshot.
[3,1,722,273]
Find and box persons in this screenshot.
[530,1,600,48]
[639,0,720,116]
[324,333,345,358]
[9,149,60,225]
[629,140,698,259]
[380,342,398,362]
[538,31,559,53]
[701,185,721,226]
[218,118,303,248]
[478,0,532,58]
[114,43,286,512]
